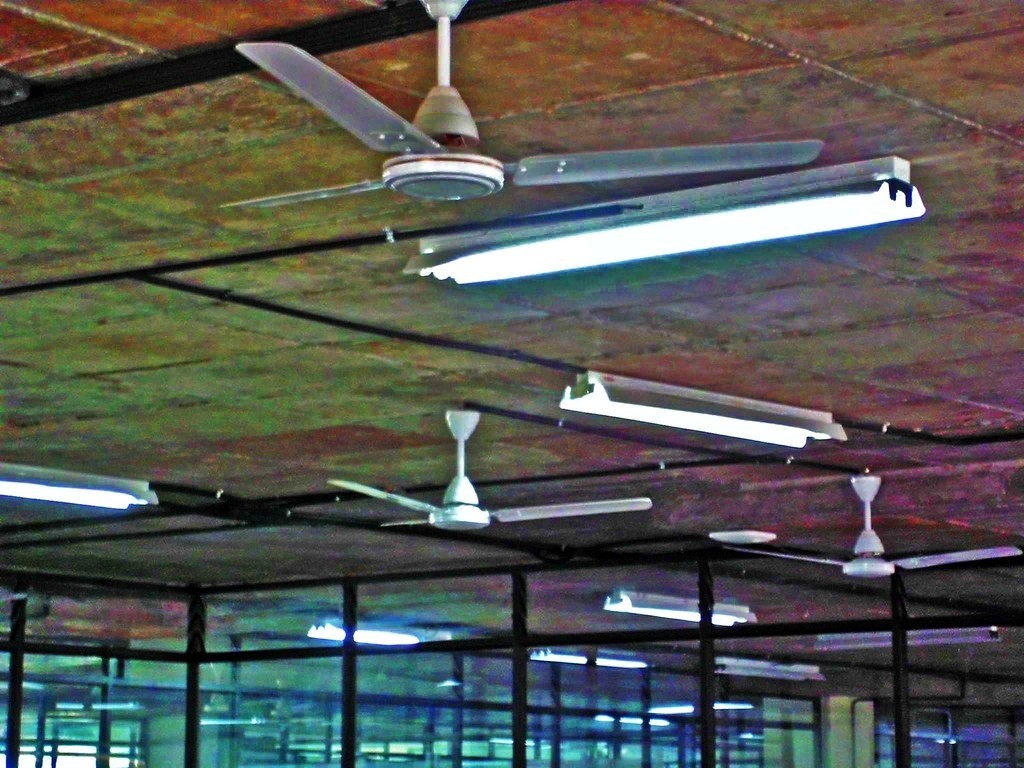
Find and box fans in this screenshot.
[325,410,653,530]
[722,475,1024,577]
[216,0,826,213]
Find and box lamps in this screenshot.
[53,589,1001,729]
[0,461,160,510]
[400,155,927,286]
[557,369,849,449]
